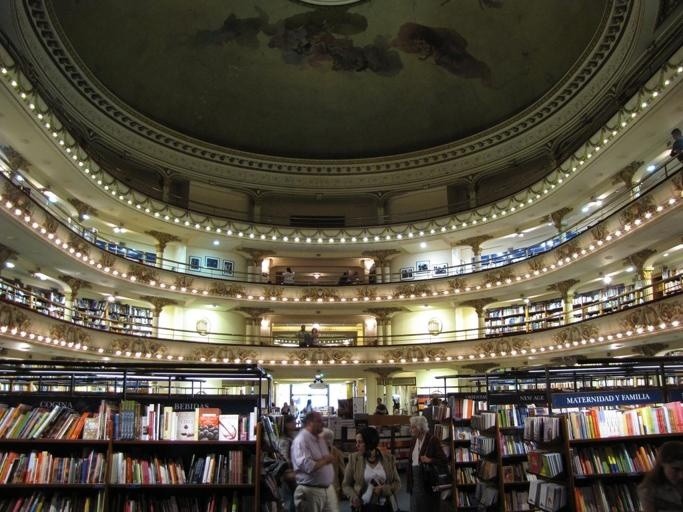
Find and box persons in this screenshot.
[670,128,683,163]
[270,397,449,511]
[336,267,376,285]
[297,325,310,347]
[281,267,295,284]
[310,328,319,346]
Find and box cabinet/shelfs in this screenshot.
[0,274,155,338]
[483,267,682,338]
[406,365,683,511]
[0,360,277,512]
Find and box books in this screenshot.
[429,397,683,511]
[1,399,257,511]
[485,267,683,337]
[0,275,153,337]
[257,397,414,472]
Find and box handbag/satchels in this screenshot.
[423,462,448,486]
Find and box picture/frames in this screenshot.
[400,260,448,281]
[188,255,235,278]
